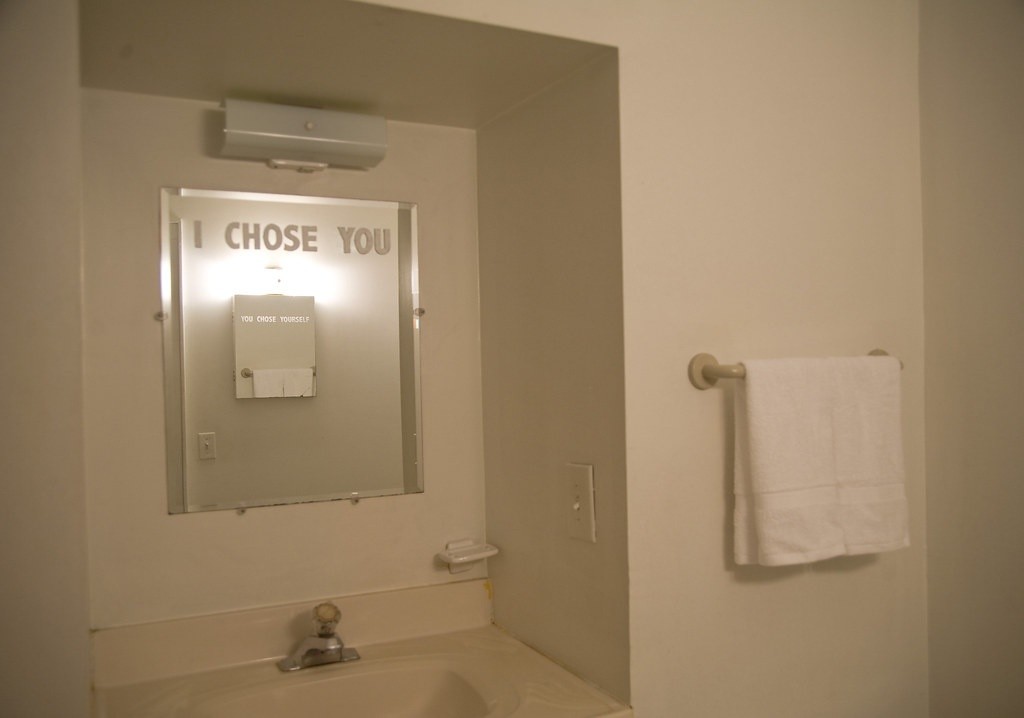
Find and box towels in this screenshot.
[732,355,911,567]
[253,368,313,399]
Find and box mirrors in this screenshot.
[159,186,424,515]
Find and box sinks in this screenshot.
[87,577,634,718]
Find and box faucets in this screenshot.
[275,601,361,672]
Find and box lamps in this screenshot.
[215,96,390,174]
